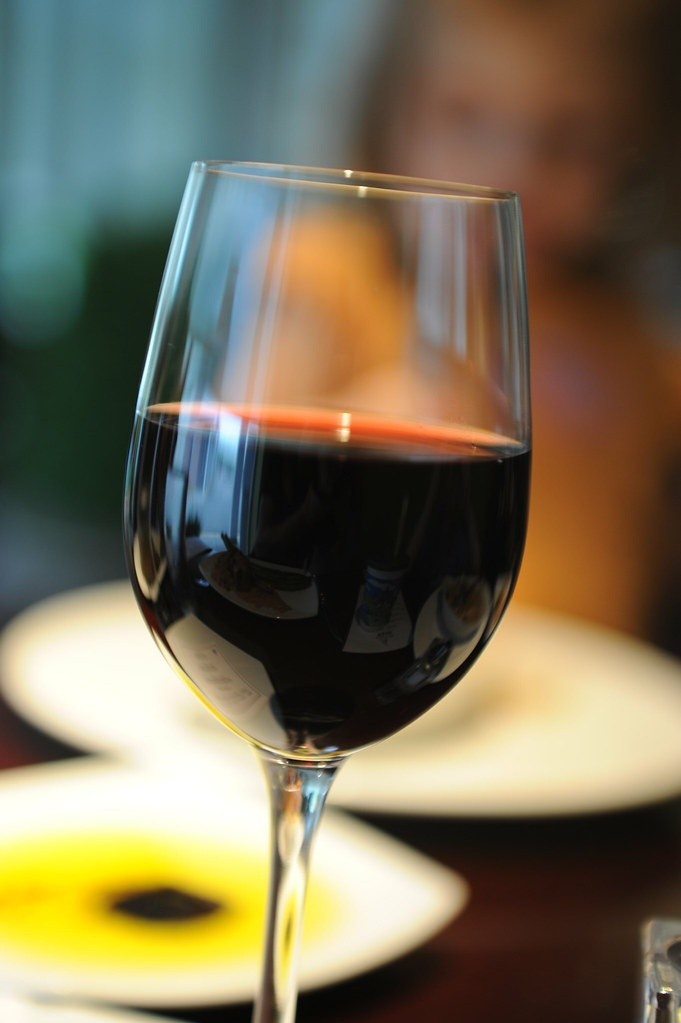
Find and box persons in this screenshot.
[245,0,680,633]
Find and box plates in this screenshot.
[1,575,680,821]
[1,768,468,1008]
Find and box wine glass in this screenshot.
[126,159,529,1023]
